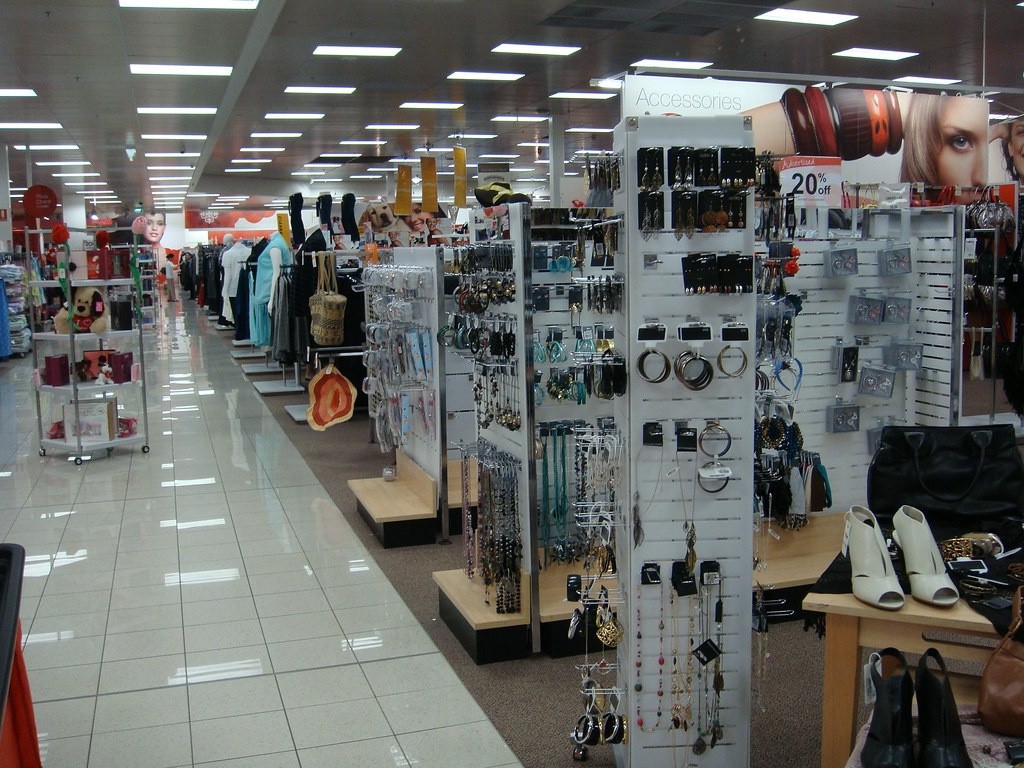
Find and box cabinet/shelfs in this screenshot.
[111,244,159,330]
[0,253,30,357]
[23,223,149,464]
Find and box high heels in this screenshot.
[892,505,959,605]
[843,505,904,611]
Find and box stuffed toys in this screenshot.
[53,287,108,334]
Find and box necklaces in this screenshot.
[667,728,698,768]
[474,365,520,435]
[669,582,696,731]
[458,445,523,614]
[675,444,698,571]
[637,577,665,732]
[693,574,723,755]
[633,442,664,549]
[539,426,588,569]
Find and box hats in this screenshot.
[305,363,357,430]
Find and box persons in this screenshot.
[166,254,180,302]
[398,203,447,232]
[737,82,988,195]
[186,252,196,300]
[140,211,183,283]
[988,121,1024,185]
[218,231,289,348]
[332,218,450,251]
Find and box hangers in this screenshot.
[279,262,296,277]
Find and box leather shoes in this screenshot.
[915,647,974,768]
[858,644,915,768]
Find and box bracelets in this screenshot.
[532,340,616,363]
[673,350,713,391]
[576,715,594,744]
[436,325,515,357]
[755,417,804,453]
[621,715,631,745]
[779,83,902,166]
[601,712,619,741]
[718,346,747,378]
[597,717,606,745]
[697,462,729,494]
[636,349,671,383]
[699,425,732,458]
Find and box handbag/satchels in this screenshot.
[911,182,1016,383]
[309,251,347,344]
[977,585,1024,739]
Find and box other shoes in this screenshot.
[167,299,179,302]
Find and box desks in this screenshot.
[802,586,1003,767]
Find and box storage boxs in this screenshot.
[43,247,131,443]
